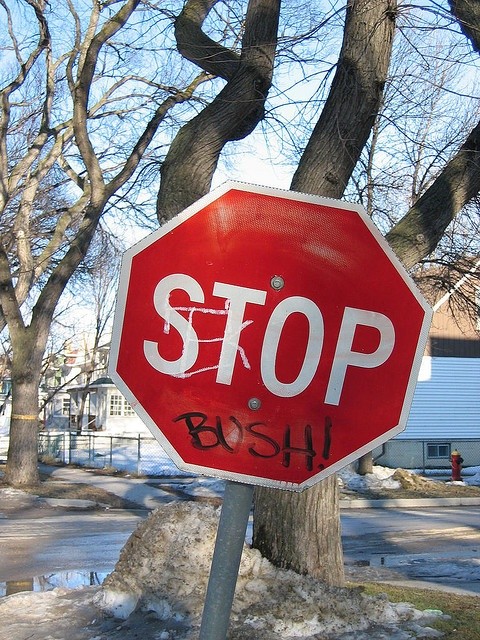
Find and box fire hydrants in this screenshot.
[450,448,463,481]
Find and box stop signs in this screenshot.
[106,178,433,494]
